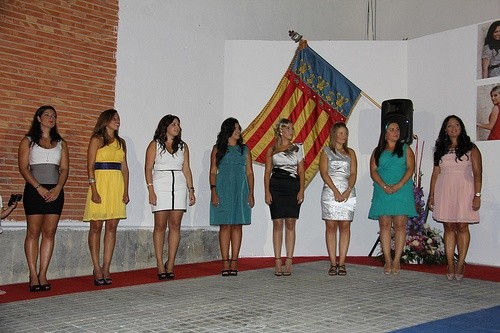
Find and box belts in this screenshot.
[488,63,500,69]
[94,162,122,170]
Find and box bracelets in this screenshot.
[146,183,153,188]
[211,185,216,188]
[475,193,481,197]
[36,185,40,189]
[190,187,194,191]
[89,178,95,183]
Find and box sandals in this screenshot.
[338,265,346,276]
[328,263,338,276]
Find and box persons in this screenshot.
[209,118,255,275]
[264,118,306,277]
[477,86,500,140]
[83,109,130,285]
[482,20,500,78]
[368,120,419,275]
[18,106,69,292]
[319,122,357,275]
[428,115,482,280]
[145,114,196,280]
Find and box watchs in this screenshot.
[383,185,387,189]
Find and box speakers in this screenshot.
[381,99,413,146]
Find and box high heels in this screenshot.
[391,263,400,276]
[229,259,239,276]
[282,258,293,276]
[102,273,112,284]
[383,263,391,275]
[38,274,50,290]
[220,259,231,276]
[158,273,167,280]
[274,258,283,275]
[30,277,40,290]
[93,270,104,285]
[164,261,174,277]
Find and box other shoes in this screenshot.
[445,273,454,281]
[455,272,463,282]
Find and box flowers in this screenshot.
[374,137,467,274]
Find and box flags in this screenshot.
[241,43,361,189]
